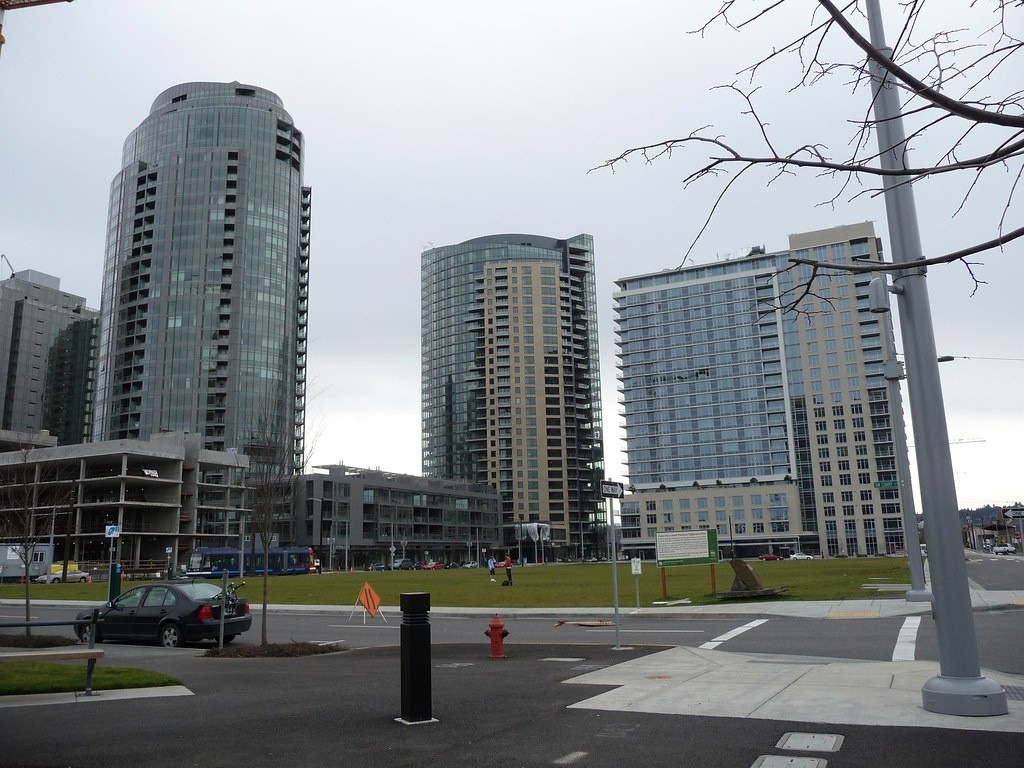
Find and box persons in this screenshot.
[502,554,512,586]
[488,556,496,582]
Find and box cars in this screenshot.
[74,581,252,647]
[410,562,458,570]
[759,554,783,561]
[790,553,814,560]
[495,562,512,567]
[37,569,90,583]
[463,561,477,568]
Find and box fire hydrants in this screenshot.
[484,613,510,658]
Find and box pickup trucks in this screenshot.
[994,543,1016,555]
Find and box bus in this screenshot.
[121,570,163,581]
[186,547,310,575]
[309,548,316,573]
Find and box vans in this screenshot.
[920,544,928,556]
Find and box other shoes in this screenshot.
[491,579,496,582]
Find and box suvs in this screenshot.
[368,563,386,569]
[389,559,410,570]
